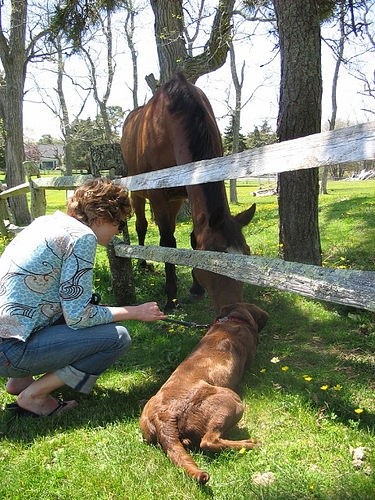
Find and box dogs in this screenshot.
[139,302,269,486]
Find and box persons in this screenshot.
[0,176,169,419]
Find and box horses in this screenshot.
[120,70,257,316]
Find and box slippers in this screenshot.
[5,403,20,412]
[18,399,78,418]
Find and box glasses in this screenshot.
[116,218,126,231]
